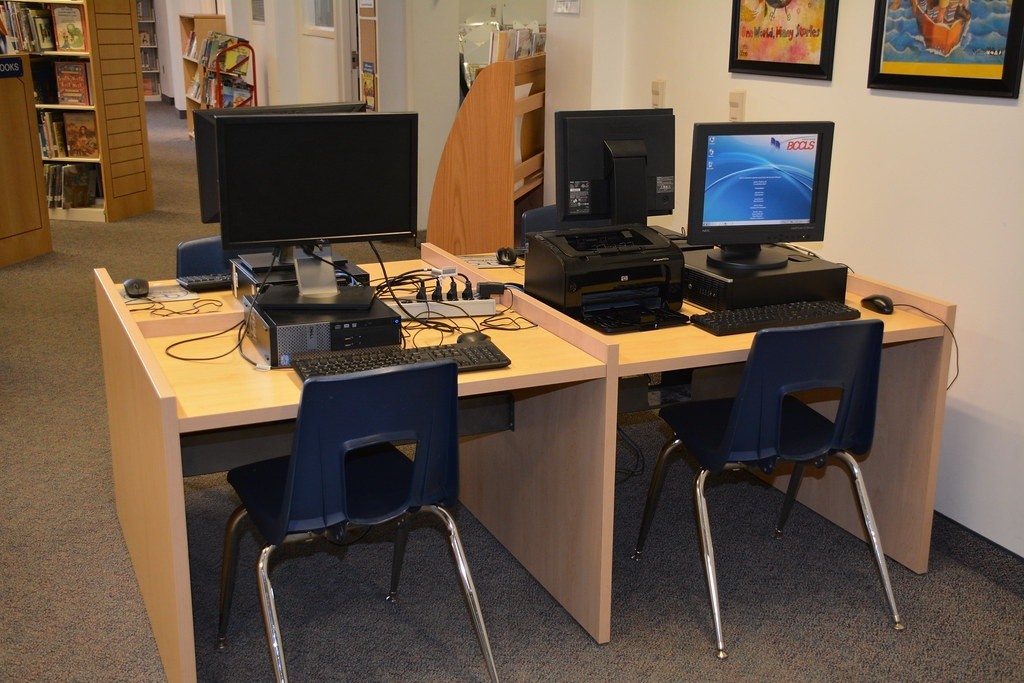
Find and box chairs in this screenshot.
[521,203,613,251]
[215,359,499,683]
[630,319,904,658]
[176,236,278,281]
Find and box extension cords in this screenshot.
[383,295,495,320]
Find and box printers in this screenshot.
[524,227,690,336]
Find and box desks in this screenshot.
[94,242,958,682]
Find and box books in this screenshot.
[37,111,99,159]
[489,29,546,64]
[0,0,89,54]
[44,162,97,210]
[55,61,89,105]
[184,30,252,108]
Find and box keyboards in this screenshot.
[690,300,861,337]
[177,273,233,291]
[288,341,512,384]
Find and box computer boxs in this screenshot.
[667,239,848,312]
[227,259,401,370]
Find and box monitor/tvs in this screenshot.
[555,107,675,226]
[192,101,419,272]
[688,121,835,269]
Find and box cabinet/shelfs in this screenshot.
[425,49,549,254]
[181,13,256,140]
[0,0,163,268]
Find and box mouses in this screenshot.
[122,279,150,298]
[457,332,491,344]
[861,294,894,315]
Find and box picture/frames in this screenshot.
[728,1,838,80]
[866,0,1024,97]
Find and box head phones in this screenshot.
[496,247,517,264]
